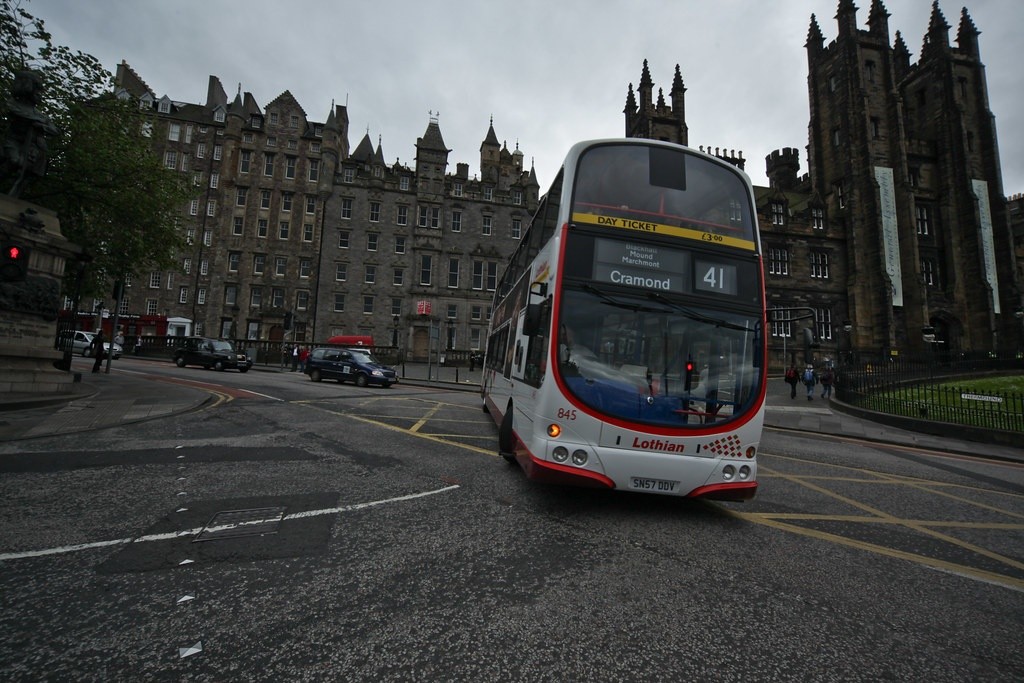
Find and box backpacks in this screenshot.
[805,369,814,381]
[785,368,795,384]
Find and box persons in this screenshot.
[114,331,124,346]
[89,330,107,374]
[801,364,819,400]
[281,343,309,374]
[785,364,800,399]
[821,367,835,400]
[558,325,599,365]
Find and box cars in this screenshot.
[302,347,400,389]
[57,328,123,360]
[171,335,254,373]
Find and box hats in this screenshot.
[807,364,813,369]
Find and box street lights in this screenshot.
[310,183,332,350]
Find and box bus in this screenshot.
[477,136,824,502]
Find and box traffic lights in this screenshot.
[0,241,32,283]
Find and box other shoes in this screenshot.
[791,393,797,399]
[821,393,825,399]
[807,395,813,401]
